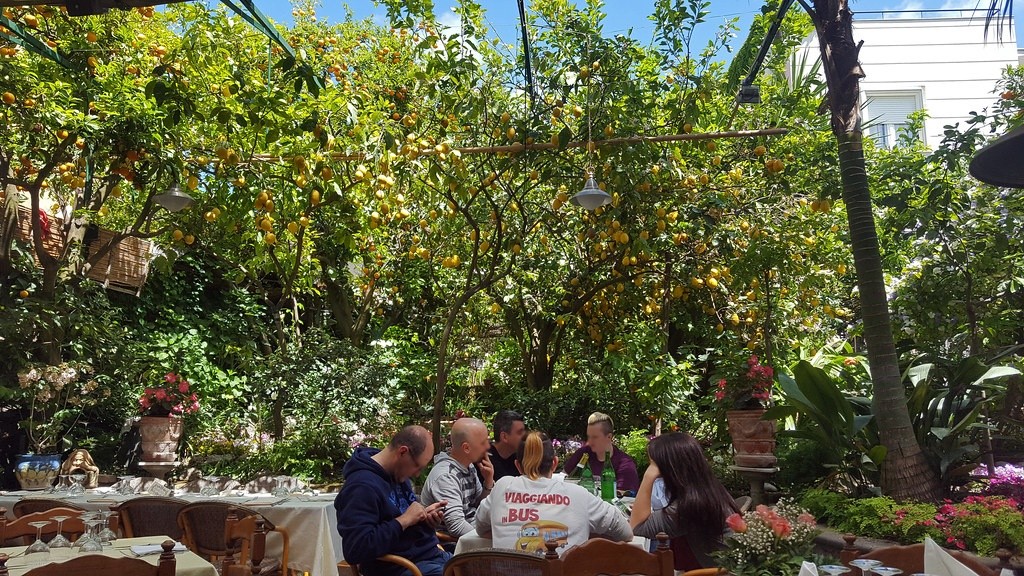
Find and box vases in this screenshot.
[15,452,64,490]
[725,409,778,468]
[138,416,184,462]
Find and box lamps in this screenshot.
[735,82,761,105]
[150,162,196,212]
[571,34,614,211]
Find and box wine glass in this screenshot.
[44,473,315,500]
[24,511,118,562]
[818,560,937,576]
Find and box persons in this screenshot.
[630,431,744,576]
[474,431,633,560]
[473,409,529,483]
[334,424,455,576]
[420,419,495,554]
[558,412,640,494]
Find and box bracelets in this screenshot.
[482,480,495,492]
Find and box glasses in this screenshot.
[401,442,428,474]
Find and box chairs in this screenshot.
[0,532,1014,576]
[176,500,290,576]
[12,499,109,547]
[0,507,119,548]
[108,494,209,563]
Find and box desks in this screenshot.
[452,510,651,554]
[0,484,345,576]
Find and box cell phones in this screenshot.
[428,506,446,515]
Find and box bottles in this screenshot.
[578,462,594,494]
[568,452,590,478]
[600,451,617,504]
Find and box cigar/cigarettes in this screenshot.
[486,452,493,456]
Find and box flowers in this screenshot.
[16,359,112,455]
[708,351,776,409]
[136,370,201,419]
[703,496,842,576]
